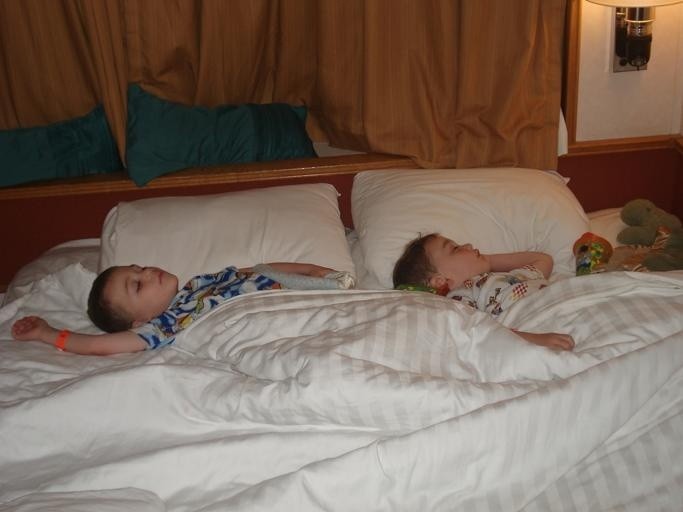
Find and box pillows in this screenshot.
[349,167,597,284]
[98,182,356,287]
[0,103,124,190]
[125,79,320,188]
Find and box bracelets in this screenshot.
[54,329,72,352]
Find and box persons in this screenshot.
[391,232,576,353]
[10,261,355,355]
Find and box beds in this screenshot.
[0,206,683,512]
